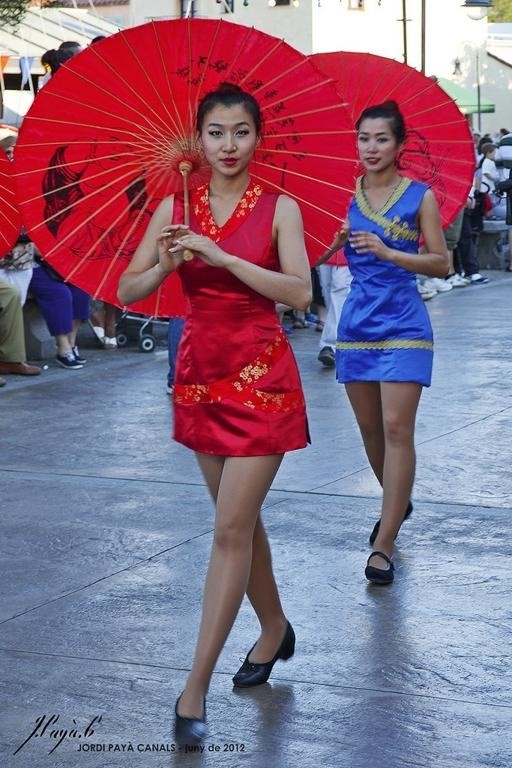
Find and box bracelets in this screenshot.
[155,264,169,276]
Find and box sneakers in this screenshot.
[54,354,83,369]
[305,312,318,326]
[282,326,294,337]
[369,498,413,543]
[364,551,396,584]
[68,345,86,364]
[317,345,336,367]
[417,271,489,301]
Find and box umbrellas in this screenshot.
[12,15,356,320]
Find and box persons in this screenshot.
[116,83,314,746]
[1,36,511,386]
[318,101,451,582]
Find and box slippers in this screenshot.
[316,320,325,332]
[103,334,118,351]
[87,317,106,346]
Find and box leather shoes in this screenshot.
[0,361,42,376]
[172,688,209,747]
[233,619,296,688]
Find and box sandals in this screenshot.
[293,316,309,329]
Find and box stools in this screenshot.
[476,218,507,266]
[21,291,59,362]
